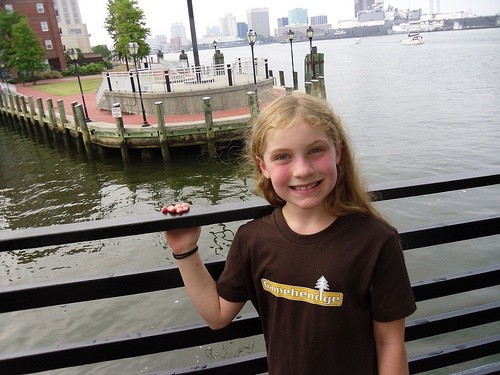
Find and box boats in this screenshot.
[401,33,424,45]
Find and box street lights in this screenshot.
[287,29,296,90]
[246,30,261,115]
[306,25,314,53]
[212,39,217,51]
[69,47,92,122]
[127,40,150,127]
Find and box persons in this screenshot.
[161,91,417,375]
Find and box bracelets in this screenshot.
[171,246,198,259]
